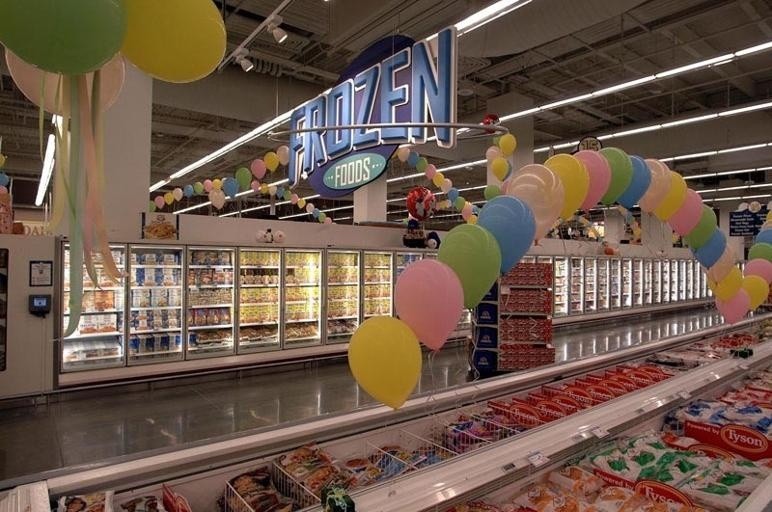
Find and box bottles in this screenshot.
[51,232,749,390]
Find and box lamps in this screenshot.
[234,18,288,72]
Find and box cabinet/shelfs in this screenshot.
[467,262,556,377]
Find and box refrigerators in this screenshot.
[1,311,772,512]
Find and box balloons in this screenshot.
[348,224,501,410]
[478,164,562,276]
[0,0,124,79]
[150,143,336,224]
[688,204,771,323]
[399,148,477,223]
[1,154,15,233]
[118,0,227,84]
[484,133,516,199]
[544,147,704,243]
[4,48,129,124]
